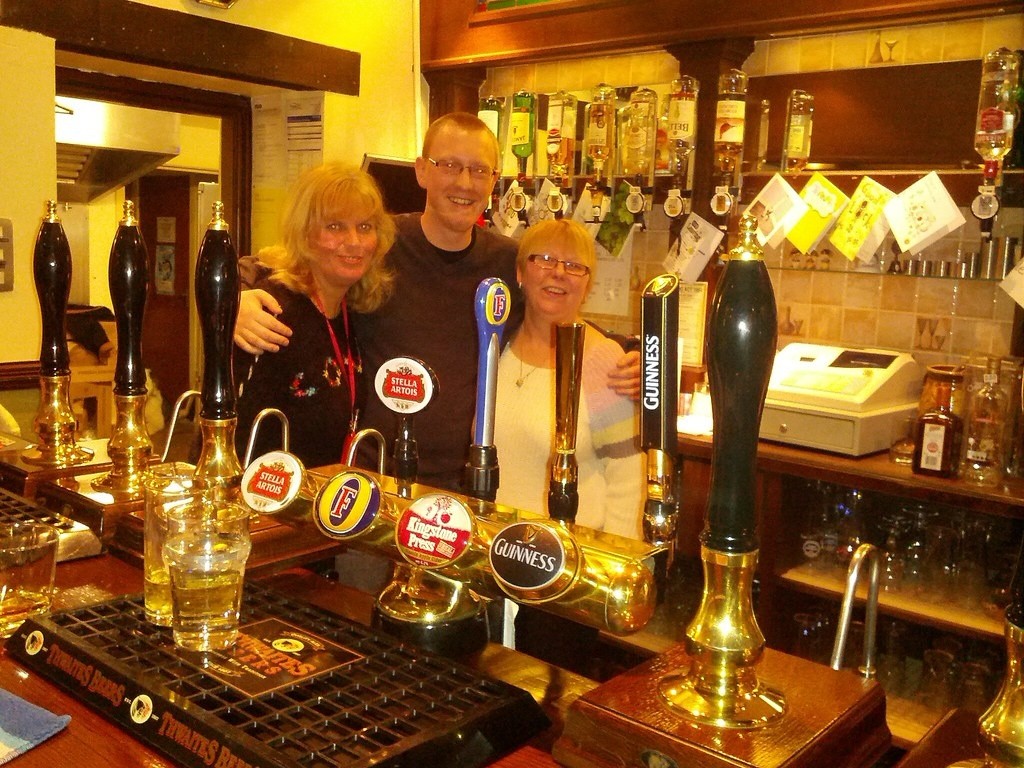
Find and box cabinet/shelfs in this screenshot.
[765,460,1024,750]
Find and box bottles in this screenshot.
[666,75,700,190]
[912,385,963,478]
[963,357,1009,487]
[477,98,500,142]
[510,91,534,182]
[916,363,970,424]
[713,68,749,186]
[782,90,814,186]
[779,306,794,335]
[545,83,657,186]
[974,51,1019,186]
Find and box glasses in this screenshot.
[524,253,591,278]
[427,156,496,181]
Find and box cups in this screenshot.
[143,465,252,650]
[888,413,918,464]
[935,335,945,349]
[794,320,803,333]
[0,521,59,638]
[901,235,1017,280]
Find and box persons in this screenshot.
[186,162,398,472]
[472,219,648,683]
[232,112,641,494]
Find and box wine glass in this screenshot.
[916,318,938,349]
[792,480,1017,722]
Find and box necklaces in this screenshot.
[516,320,556,387]
